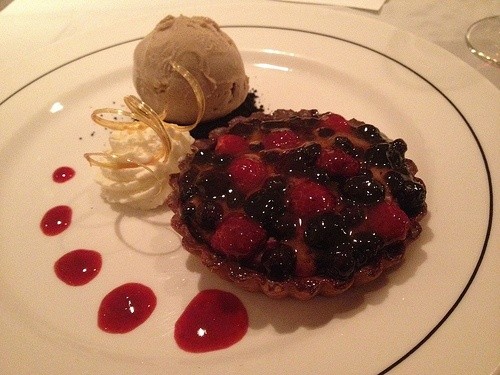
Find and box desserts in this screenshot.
[164,108,428,302]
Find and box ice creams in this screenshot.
[86,12,249,210]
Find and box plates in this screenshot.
[0,1,500,375]
[465,15,500,65]
